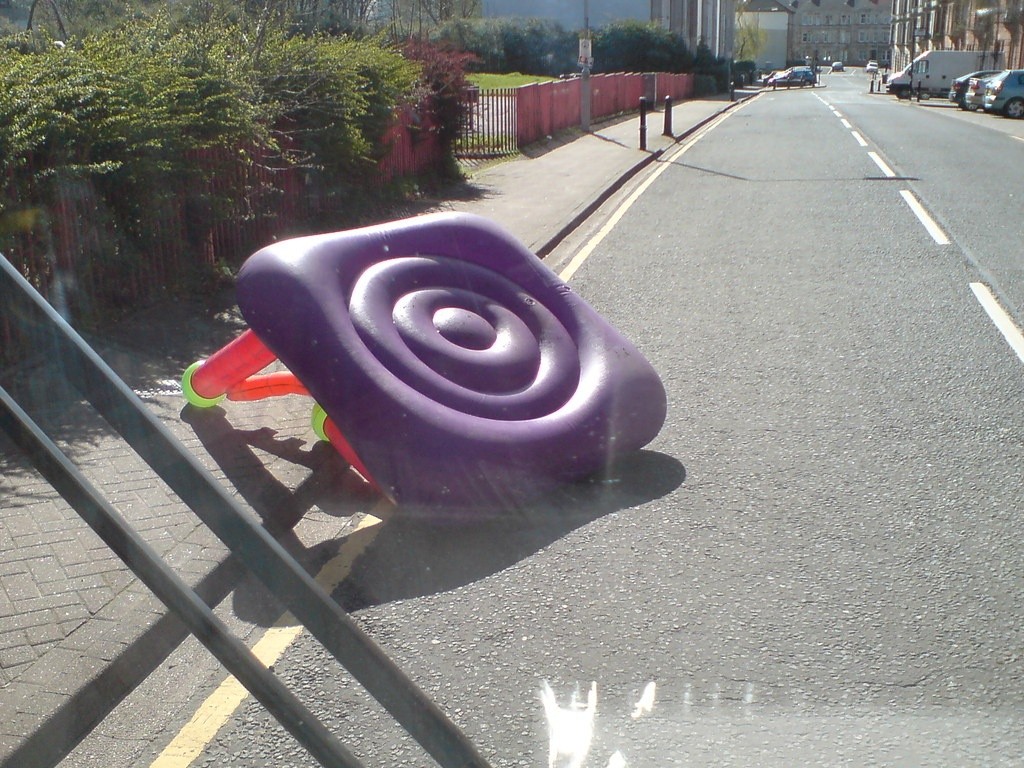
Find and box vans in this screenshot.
[886,50,1006,100]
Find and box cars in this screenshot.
[866,62,879,73]
[948,69,992,110]
[763,63,818,88]
[965,72,1002,107]
[982,69,1024,119]
[832,61,844,72]
